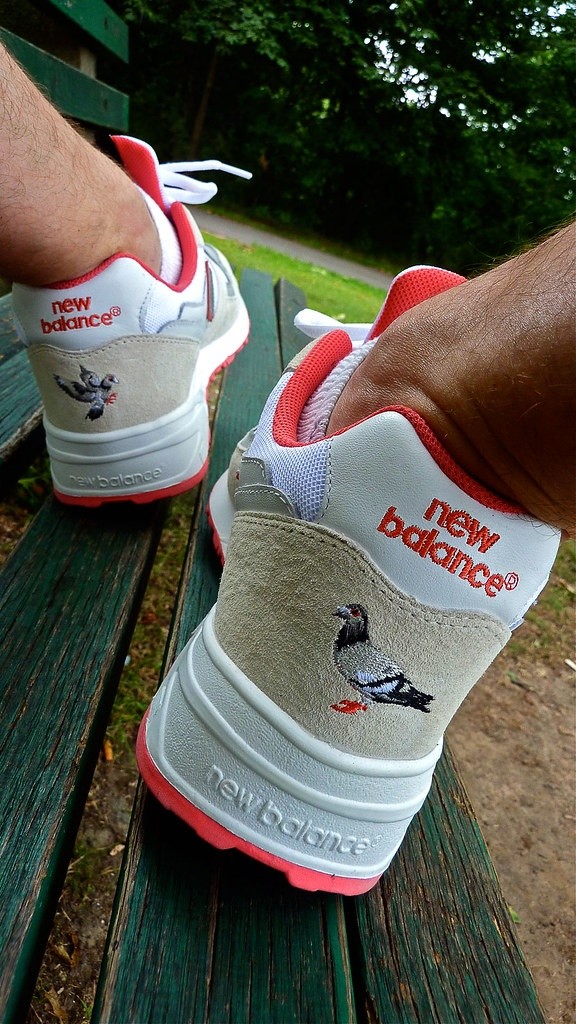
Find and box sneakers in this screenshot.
[11,135,252,507]
[137,265,562,895]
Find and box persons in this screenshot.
[0,42,576,897]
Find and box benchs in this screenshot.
[0,0,541,1024]
